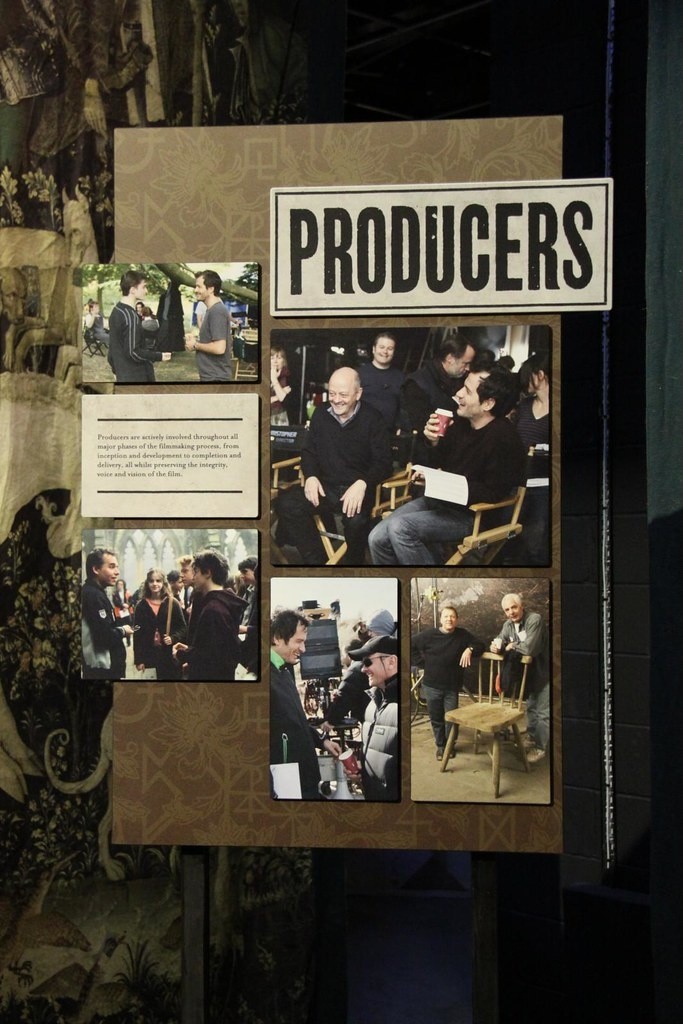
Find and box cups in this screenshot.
[338,750,358,772]
[432,408,453,435]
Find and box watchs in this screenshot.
[469,646,475,651]
[193,344,196,350]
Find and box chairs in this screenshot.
[230,328,258,381]
[83,327,109,357]
[439,651,532,800]
[272,426,417,566]
[382,447,535,565]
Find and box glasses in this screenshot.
[361,656,390,667]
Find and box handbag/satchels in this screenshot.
[166,645,184,673]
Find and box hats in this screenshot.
[366,608,396,636]
[348,636,397,661]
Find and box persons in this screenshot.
[84,269,243,383]
[82,548,259,682]
[270,332,553,567]
[411,593,549,764]
[269,609,401,801]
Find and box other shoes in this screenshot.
[526,748,545,762]
[436,747,455,760]
[515,735,536,748]
